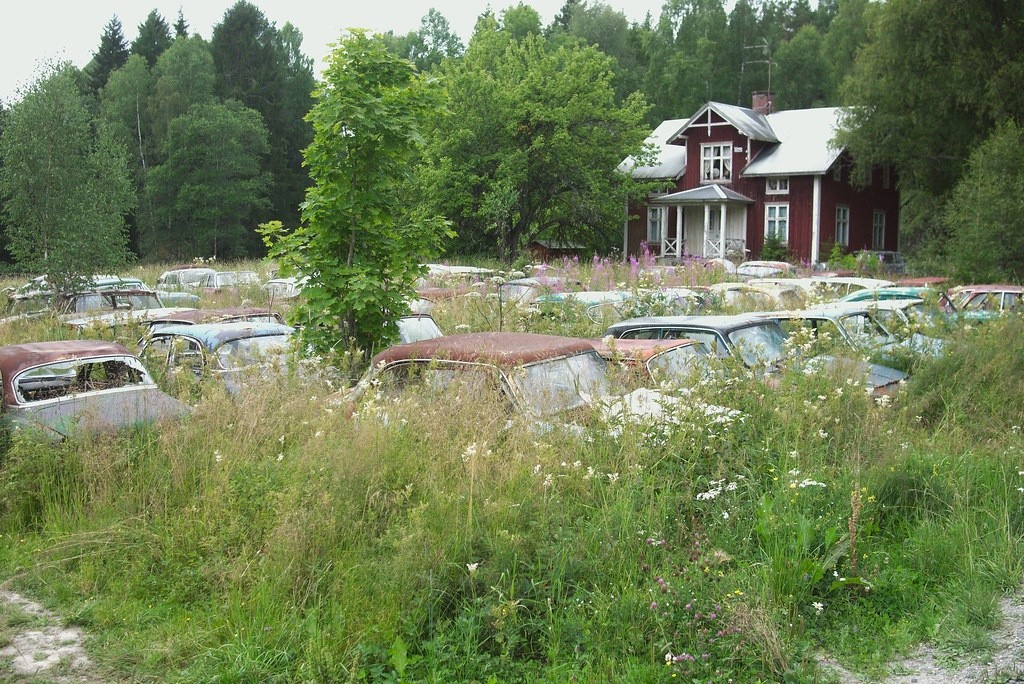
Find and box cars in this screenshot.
[0,254,1023,467]
